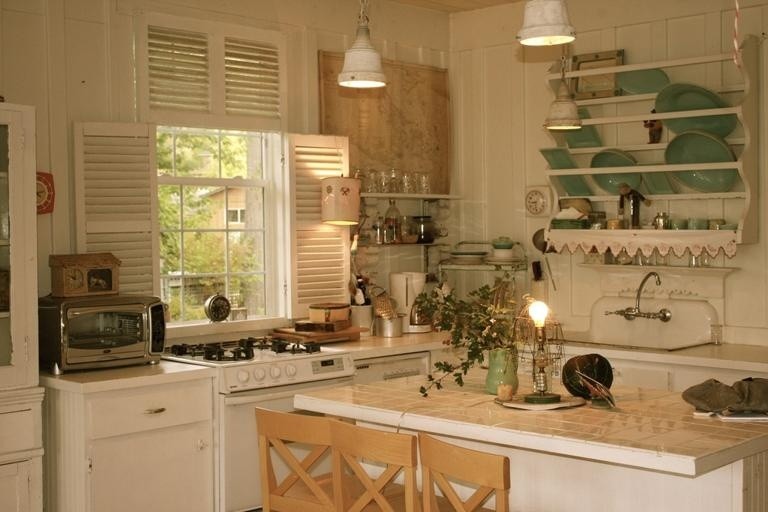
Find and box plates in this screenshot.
[481,257,523,262]
[538,109,596,197]
[615,67,738,137]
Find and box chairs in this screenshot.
[329,418,497,512]
[418,429,510,512]
[254,406,405,512]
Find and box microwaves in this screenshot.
[39,296,167,377]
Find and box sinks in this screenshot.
[542,331,669,387]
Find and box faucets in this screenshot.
[635,271,660,311]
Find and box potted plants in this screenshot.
[416,264,529,398]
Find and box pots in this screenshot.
[309,303,350,323]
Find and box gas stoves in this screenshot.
[161,336,357,394]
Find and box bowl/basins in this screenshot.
[447,251,488,266]
[589,132,736,195]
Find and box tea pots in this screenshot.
[654,212,670,229]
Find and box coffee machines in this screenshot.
[389,271,432,334]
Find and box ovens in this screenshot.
[215,379,355,512]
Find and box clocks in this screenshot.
[525,190,545,215]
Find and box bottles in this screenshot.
[374,198,436,245]
[355,274,369,306]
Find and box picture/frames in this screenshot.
[569,48,625,102]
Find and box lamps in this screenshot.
[337,0,387,89]
[544,43,583,131]
[504,294,569,403]
[319,176,363,226]
[516,0,577,47]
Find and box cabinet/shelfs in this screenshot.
[357,191,465,248]
[39,367,221,512]
[0,99,48,512]
[544,34,761,258]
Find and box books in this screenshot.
[691,405,768,421]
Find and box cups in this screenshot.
[634,249,710,266]
[711,324,723,347]
[353,168,430,193]
